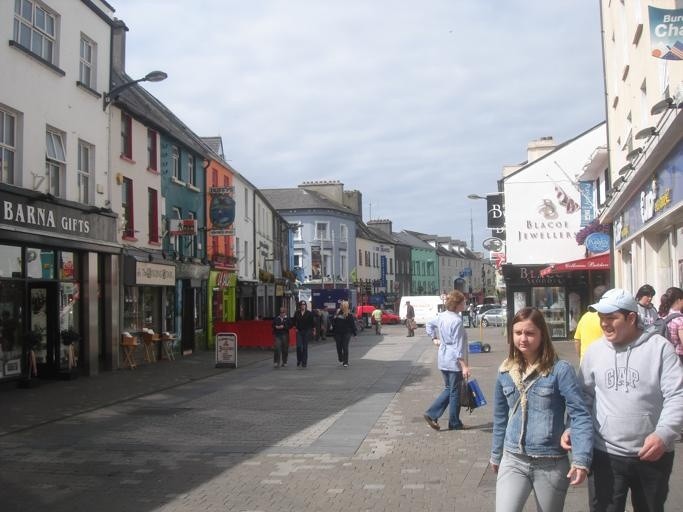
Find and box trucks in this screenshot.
[398,295,461,326]
[310,288,357,330]
[354,305,400,325]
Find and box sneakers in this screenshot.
[449,424,472,430]
[424,415,440,431]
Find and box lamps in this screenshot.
[102,71,167,112]
[600,98,676,208]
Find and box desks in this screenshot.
[121,332,176,369]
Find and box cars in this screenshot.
[464,302,506,327]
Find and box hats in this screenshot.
[587,288,638,313]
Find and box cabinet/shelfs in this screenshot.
[537,306,565,338]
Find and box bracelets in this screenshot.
[432,337,437,340]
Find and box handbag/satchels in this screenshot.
[371,315,376,324]
[460,382,476,413]
[469,379,487,406]
[409,320,417,330]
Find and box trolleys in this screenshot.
[479,319,491,352]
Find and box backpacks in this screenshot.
[646,313,683,346]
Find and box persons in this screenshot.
[490,306,594,512]
[272,300,358,368]
[372,306,384,336]
[426,290,471,430]
[466,303,477,327]
[561,288,683,511]
[661,287,683,360]
[574,285,609,361]
[406,301,416,336]
[635,285,659,328]
